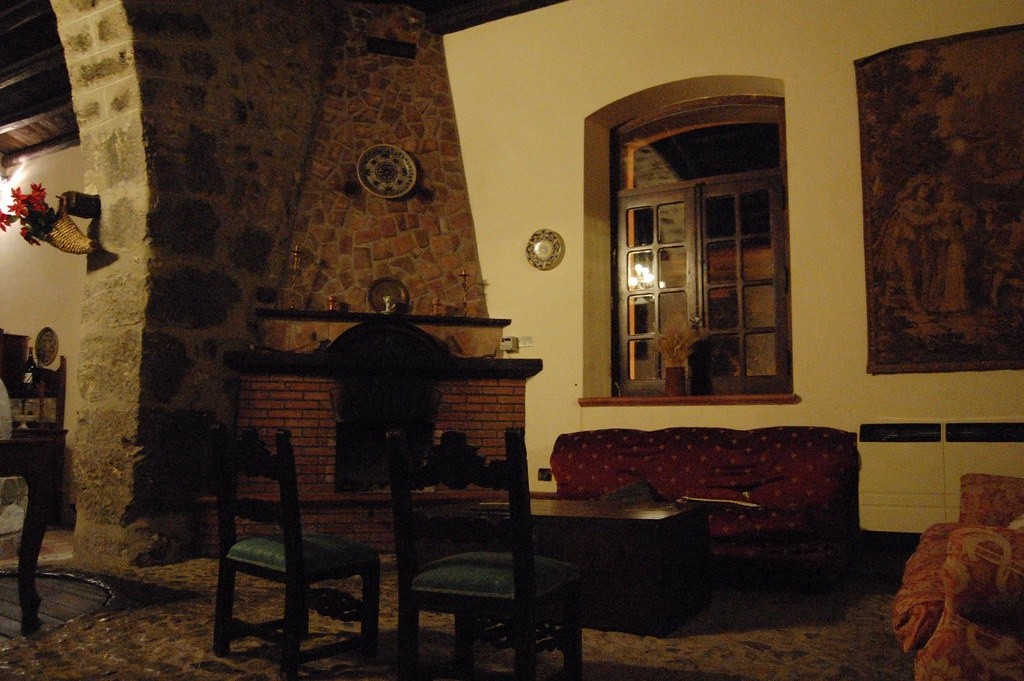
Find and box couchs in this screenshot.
[551,426,860,590]
[893,474,1024,681]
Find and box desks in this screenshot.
[1,440,55,613]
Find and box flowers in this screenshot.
[0,182,55,245]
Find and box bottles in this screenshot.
[21,346,37,397]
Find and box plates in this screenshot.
[356,144,417,199]
[526,229,565,272]
[367,277,410,314]
[35,327,58,366]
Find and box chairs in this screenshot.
[389,426,580,681]
[212,429,378,677]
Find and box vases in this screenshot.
[665,366,686,397]
[46,214,97,254]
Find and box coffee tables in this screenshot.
[409,495,714,634]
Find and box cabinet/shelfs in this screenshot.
[0,327,68,525]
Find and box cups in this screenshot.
[665,366,685,397]
